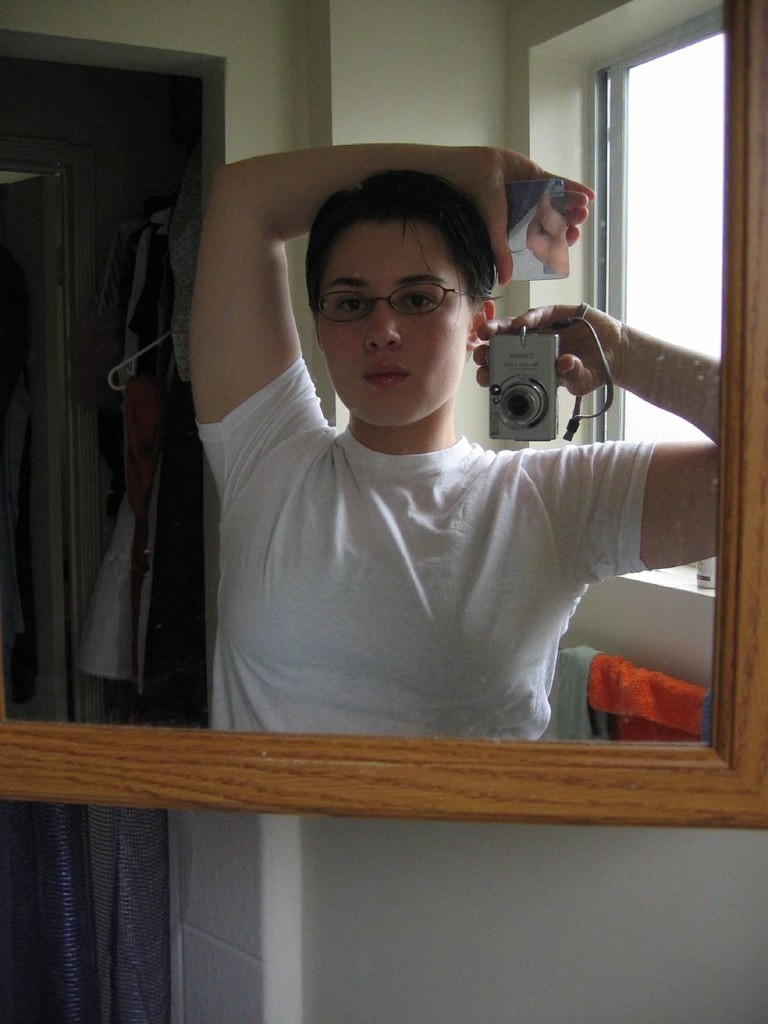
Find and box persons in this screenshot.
[189,143,721,742]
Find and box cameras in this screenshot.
[488,324,560,441]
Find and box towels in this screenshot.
[588,653,713,747]
[552,645,611,744]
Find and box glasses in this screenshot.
[311,282,472,324]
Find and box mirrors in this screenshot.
[2,0,767,836]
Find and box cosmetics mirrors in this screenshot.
[507,178,570,281]
